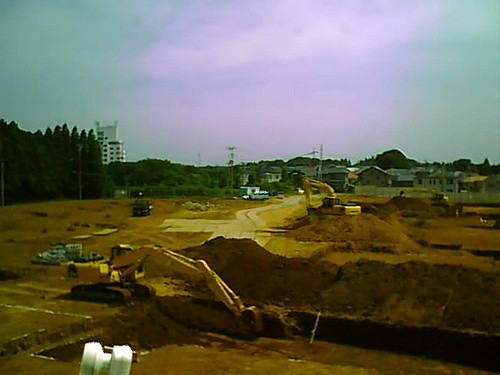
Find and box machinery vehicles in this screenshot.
[303,177,360,217]
[64,244,264,334]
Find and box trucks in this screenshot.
[132,199,153,217]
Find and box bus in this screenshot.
[250,191,269,201]
[400,186,450,206]
